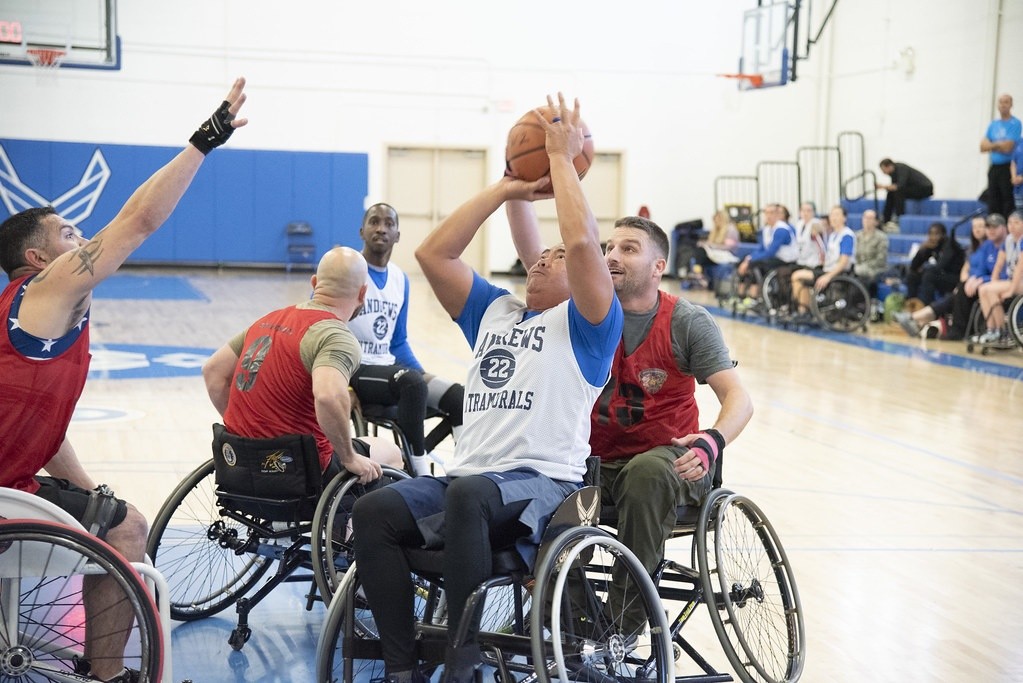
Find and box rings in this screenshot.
[553,117,560,122]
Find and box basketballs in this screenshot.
[505,105,594,193]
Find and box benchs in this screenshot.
[691,199,992,300]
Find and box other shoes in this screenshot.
[776,308,812,325]
[890,309,920,336]
[970,326,1011,343]
[594,633,639,661]
[73,654,142,683]
[326,554,356,581]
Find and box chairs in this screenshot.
[285,220,317,275]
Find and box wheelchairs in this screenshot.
[0,486,196,683]
[141,422,417,650]
[715,261,871,335]
[351,404,451,479]
[965,293,1023,355]
[312,446,810,682]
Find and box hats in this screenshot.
[984,213,1006,228]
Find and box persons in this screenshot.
[200,246,384,593]
[0,74,249,683]
[876,159,932,232]
[506,198,753,654]
[981,95,1022,225]
[310,202,467,477]
[351,90,624,683]
[1010,138,1023,211]
[677,200,1023,345]
